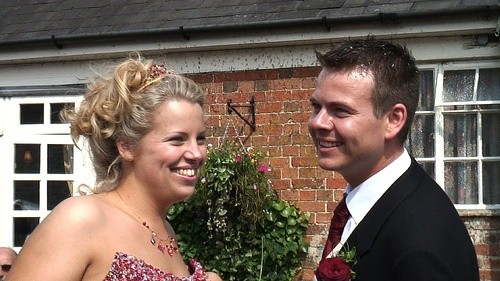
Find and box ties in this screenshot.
[322,193,351,259]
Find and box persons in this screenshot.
[0,247,17,281]
[7,51,222,281]
[308,37,479,281]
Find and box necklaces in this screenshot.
[115,186,180,255]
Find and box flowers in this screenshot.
[314,242,359,281]
